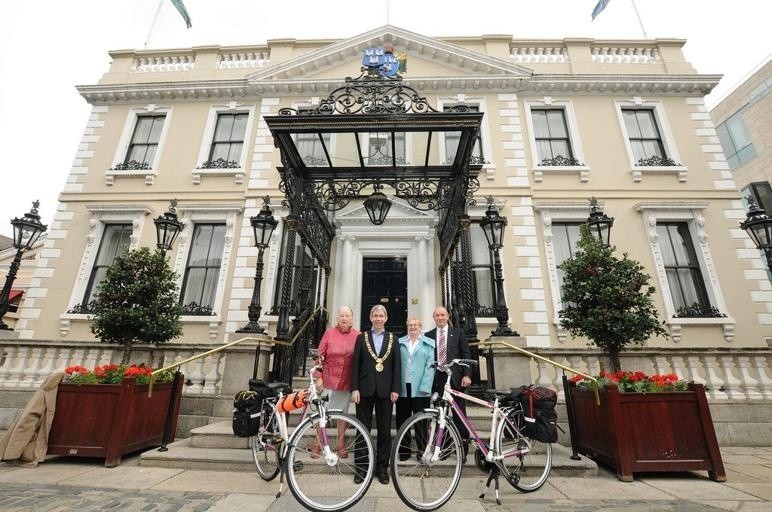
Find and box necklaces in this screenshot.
[364,332,392,373]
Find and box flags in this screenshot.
[592,0,610,21]
[170,0,193,29]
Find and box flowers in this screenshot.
[64,362,178,385]
[568,370,693,395]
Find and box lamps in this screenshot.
[362,184,393,225]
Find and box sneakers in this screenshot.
[311,444,320,459]
[338,446,347,458]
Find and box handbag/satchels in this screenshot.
[498,387,557,443]
[233,379,292,437]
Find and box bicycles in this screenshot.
[390,358,553,512]
[251,350,376,512]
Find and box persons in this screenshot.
[351,305,401,484]
[424,306,473,465]
[311,305,362,459]
[396,317,436,464]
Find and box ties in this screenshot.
[439,329,447,370]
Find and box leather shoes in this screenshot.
[376,472,389,483]
[354,472,366,483]
[400,452,466,463]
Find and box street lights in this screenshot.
[584,201,615,270]
[1,201,47,315]
[153,198,185,270]
[479,204,520,336]
[234,194,278,335]
[741,194,772,275]
